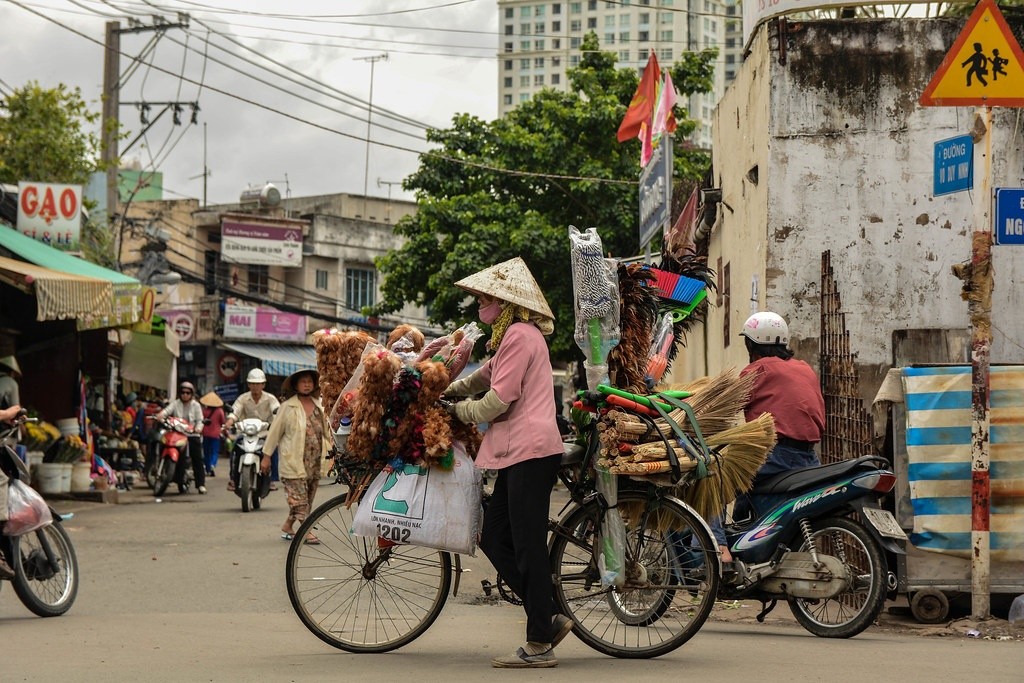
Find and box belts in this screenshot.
[776,437,816,452]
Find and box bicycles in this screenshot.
[284,396,724,661]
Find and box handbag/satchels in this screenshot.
[1,478,54,537]
[347,440,484,559]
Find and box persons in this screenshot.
[221,368,281,491]
[261,370,333,544]
[685,311,827,579]
[0,405,26,577]
[0,355,26,433]
[438,258,574,668]
[157,382,226,493]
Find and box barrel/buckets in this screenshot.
[72,463,91,492]
[60,425,80,436]
[59,464,72,492]
[37,464,62,493]
[28,452,44,464]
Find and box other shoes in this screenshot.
[210,465,216,476]
[269,483,277,491]
[199,486,207,494]
[491,646,558,668]
[689,562,739,577]
[550,613,576,648]
[226,480,236,491]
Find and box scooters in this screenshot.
[151,397,212,497]
[222,403,279,512]
[0,407,79,619]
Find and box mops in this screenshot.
[568,225,619,367]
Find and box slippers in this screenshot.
[303,537,321,544]
[281,533,296,540]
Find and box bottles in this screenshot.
[337,418,353,435]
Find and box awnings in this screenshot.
[220,341,318,375]
[0,255,114,319]
[0,224,140,283]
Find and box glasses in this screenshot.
[181,391,191,395]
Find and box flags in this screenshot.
[618,54,677,167]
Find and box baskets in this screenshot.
[332,440,377,491]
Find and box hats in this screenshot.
[200,391,224,407]
[0,355,22,377]
[454,256,557,321]
[127,392,138,403]
[281,368,320,393]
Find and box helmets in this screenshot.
[181,382,195,394]
[246,368,267,383]
[738,311,788,345]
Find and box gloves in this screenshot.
[455,389,513,426]
[442,367,492,397]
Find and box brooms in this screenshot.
[604,371,778,528]
[626,260,708,366]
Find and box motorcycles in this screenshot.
[607,454,910,639]
